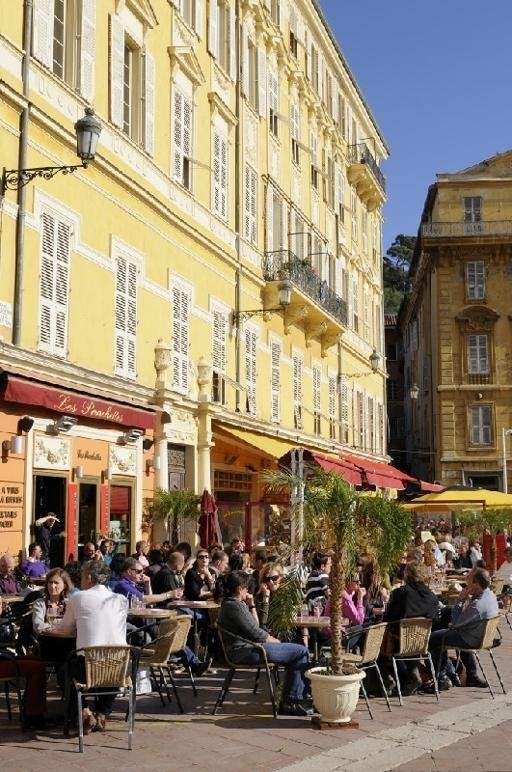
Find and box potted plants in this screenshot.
[259,463,415,725]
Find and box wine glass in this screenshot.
[46,607,57,629]
[313,602,322,622]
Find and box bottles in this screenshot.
[301,603,309,623]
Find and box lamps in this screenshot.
[232,280,292,327]
[104,468,112,479]
[124,429,143,443]
[54,416,78,433]
[338,350,381,384]
[73,466,82,477]
[147,456,160,470]
[1,109,102,198]
[4,436,22,454]
[410,382,429,400]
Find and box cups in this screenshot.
[130,594,146,613]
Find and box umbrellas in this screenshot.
[398,484,512,538]
[197,490,218,547]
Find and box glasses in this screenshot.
[264,575,279,582]
[198,555,209,559]
[132,568,143,574]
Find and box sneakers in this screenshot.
[24,716,56,731]
[278,701,308,716]
[380,672,461,695]
[83,714,106,734]
[467,674,488,688]
[195,658,212,677]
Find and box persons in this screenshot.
[35,512,68,566]
[32,567,79,727]
[295,518,511,698]
[213,571,310,716]
[51,559,129,734]
[1,597,58,734]
[251,561,296,642]
[0,535,290,681]
